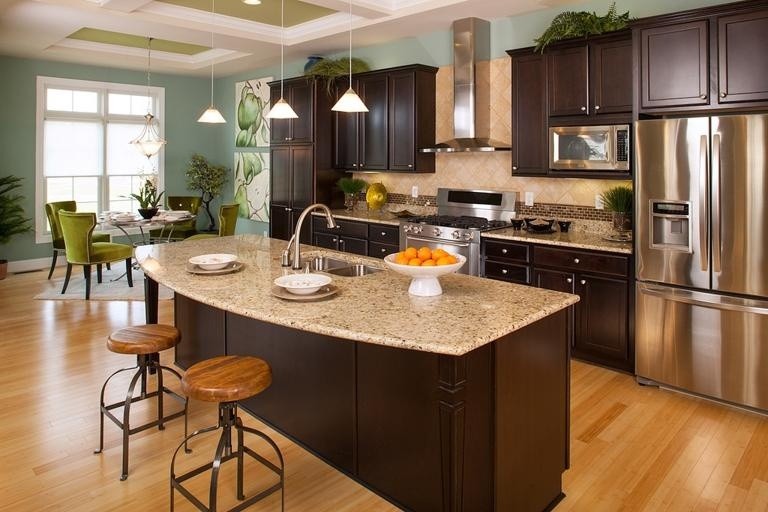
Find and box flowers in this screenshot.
[119,165,165,209]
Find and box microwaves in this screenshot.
[549,124,630,172]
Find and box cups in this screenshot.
[542,219,554,232]
[557,221,571,232]
[511,219,526,231]
[525,218,536,230]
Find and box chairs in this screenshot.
[149,196,201,244]
[94,325,193,482]
[58,209,133,300]
[183,204,240,241]
[170,355,284,512]
[44,201,111,280]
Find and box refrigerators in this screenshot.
[634,114,768,410]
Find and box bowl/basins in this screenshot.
[274,274,331,294]
[189,253,237,270]
[529,220,550,233]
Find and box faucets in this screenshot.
[282,234,295,266]
[292,204,337,269]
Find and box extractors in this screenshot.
[418,18,512,153]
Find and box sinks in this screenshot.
[294,255,352,272]
[328,266,383,277]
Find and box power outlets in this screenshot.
[411,186,418,198]
[525,191,534,205]
[594,195,605,209]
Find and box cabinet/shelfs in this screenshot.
[481,239,529,263]
[504,44,548,177]
[369,242,399,259]
[547,30,634,127]
[531,243,630,278]
[312,216,369,240]
[632,0,768,120]
[369,224,400,246]
[313,233,368,258]
[389,63,439,174]
[269,146,352,245]
[332,67,388,173]
[531,268,634,376]
[482,258,530,283]
[266,75,331,144]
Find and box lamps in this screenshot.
[129,37,167,160]
[330,0,369,113]
[265,0,299,120]
[197,1,227,124]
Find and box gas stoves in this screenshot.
[399,187,518,243]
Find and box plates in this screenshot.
[388,209,418,218]
[366,183,388,210]
[185,262,243,276]
[603,233,631,242]
[100,210,137,223]
[271,285,338,301]
[153,210,190,221]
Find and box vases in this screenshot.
[184,153,231,234]
[138,209,161,220]
[0,174,35,281]
